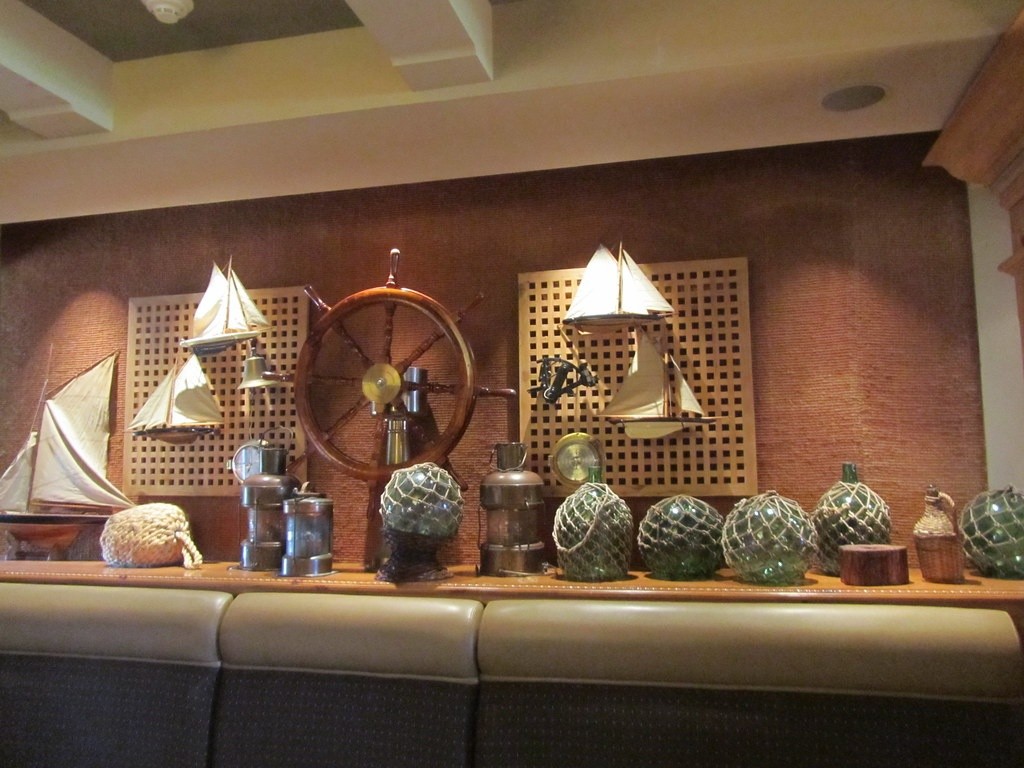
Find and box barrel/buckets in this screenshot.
[488,442,528,473]
[258,426,293,475]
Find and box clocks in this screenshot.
[230,439,273,486]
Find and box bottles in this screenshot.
[813,462,891,576]
[912,484,966,583]
[554,466,633,582]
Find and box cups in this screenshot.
[391,366,428,418]
[386,419,410,465]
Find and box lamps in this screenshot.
[240,426,301,571]
[279,481,338,577]
[477,443,548,577]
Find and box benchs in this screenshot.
[0,582,1024,768]
